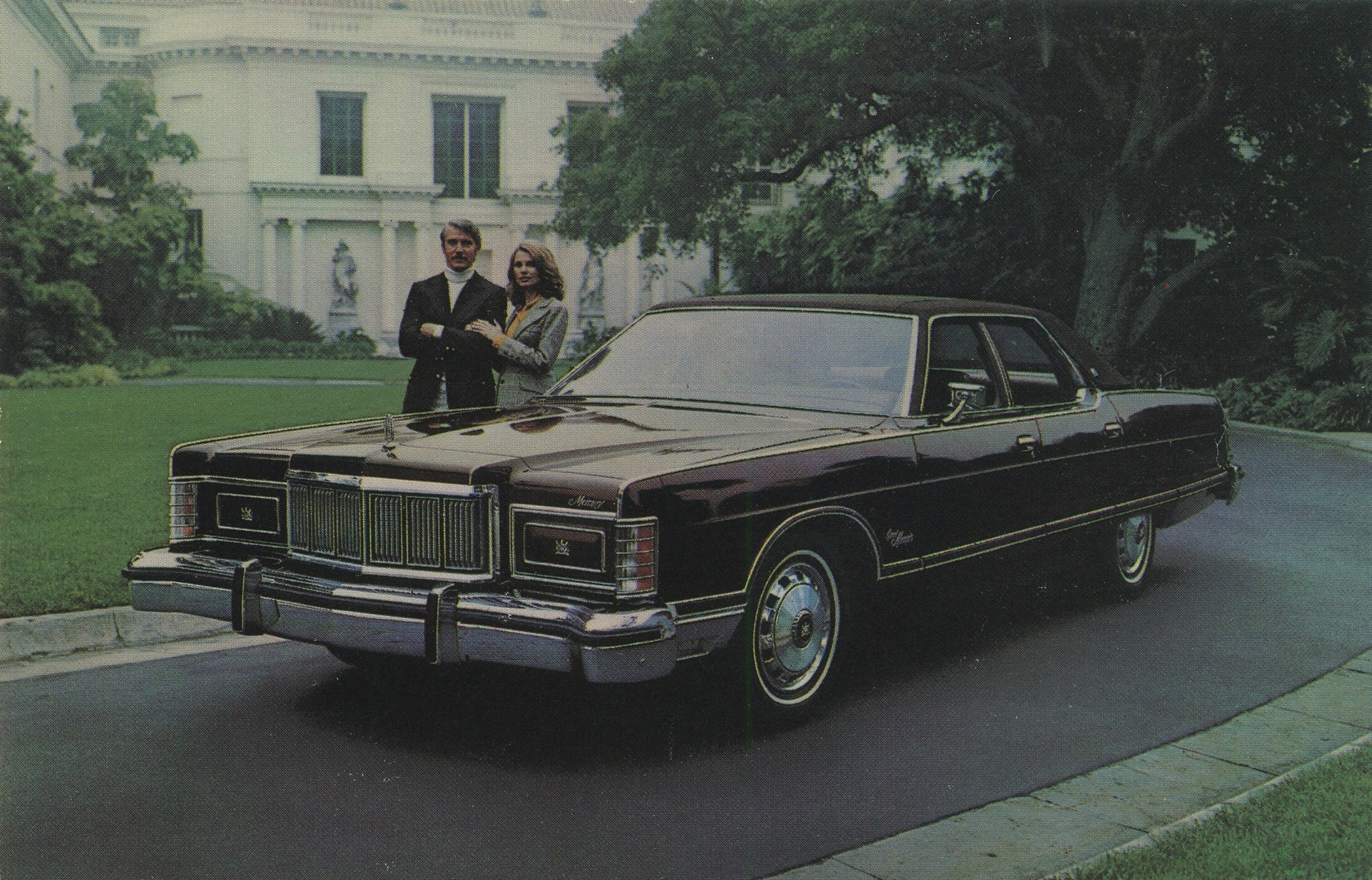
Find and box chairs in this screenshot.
[920,368,972,414]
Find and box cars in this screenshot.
[127,288,1248,728]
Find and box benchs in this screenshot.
[964,372,1072,407]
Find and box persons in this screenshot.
[576,249,605,313]
[397,217,508,414]
[329,237,360,307]
[463,238,570,408]
